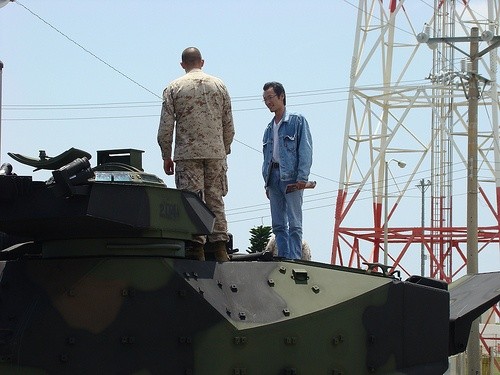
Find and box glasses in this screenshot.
[262,94,278,102]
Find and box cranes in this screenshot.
[330,0,500,374]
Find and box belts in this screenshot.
[271,162,281,168]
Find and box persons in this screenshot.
[262,80,312,260]
[265,233,312,260]
[157,48,234,264]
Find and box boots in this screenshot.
[193,242,206,261]
[213,241,231,262]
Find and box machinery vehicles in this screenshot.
[1,147,500,374]
